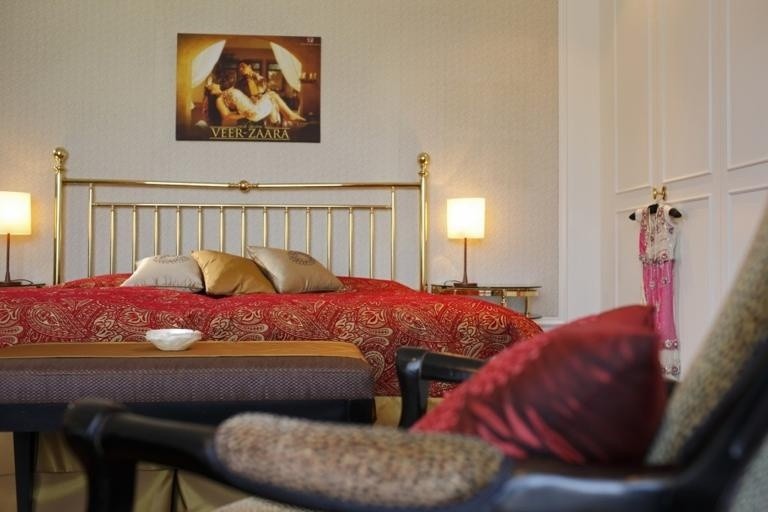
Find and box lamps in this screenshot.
[445,195,487,289]
[0,189,32,287]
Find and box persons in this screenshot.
[205,82,307,122]
[219,58,279,128]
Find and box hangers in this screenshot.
[627,189,684,221]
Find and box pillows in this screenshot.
[119,245,347,296]
[400,303,669,474]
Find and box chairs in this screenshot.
[58,196,768,512]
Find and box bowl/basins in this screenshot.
[144,328,203,352]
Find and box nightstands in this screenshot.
[430,284,545,321]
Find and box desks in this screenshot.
[0,340,376,512]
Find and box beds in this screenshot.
[0,146,545,476]
